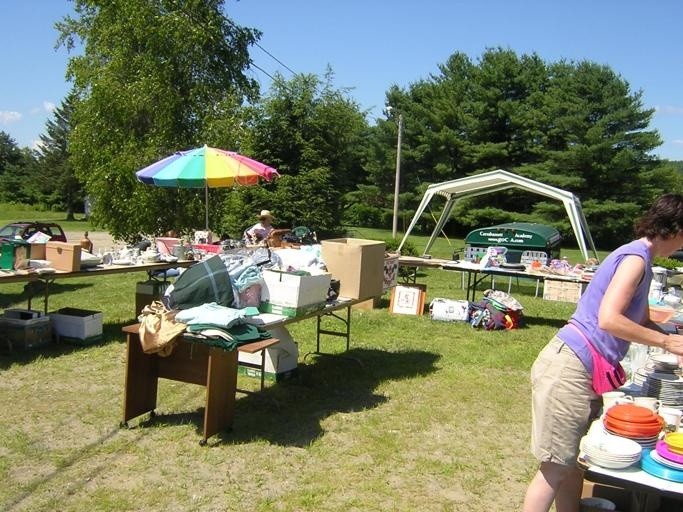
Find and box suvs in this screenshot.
[0,220,66,246]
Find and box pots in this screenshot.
[650,266,683,289]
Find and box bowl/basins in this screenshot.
[648,304,674,324]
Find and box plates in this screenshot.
[111,259,130,265]
[577,405,682,484]
[633,353,682,406]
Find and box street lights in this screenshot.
[385,105,403,240]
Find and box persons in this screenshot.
[81,231,93,254]
[133,209,275,255]
[524,193,683,512]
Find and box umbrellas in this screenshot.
[135,144,279,231]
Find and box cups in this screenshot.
[132,248,139,259]
[602,392,633,413]
[633,397,661,413]
[194,249,205,260]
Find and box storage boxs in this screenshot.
[0,308,49,353]
[352,295,382,312]
[261,266,331,318]
[320,235,385,301]
[235,336,298,383]
[45,307,102,340]
[134,292,163,322]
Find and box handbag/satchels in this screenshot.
[593,348,625,395]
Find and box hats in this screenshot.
[258,210,272,219]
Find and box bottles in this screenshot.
[186,248,193,260]
[80,231,90,252]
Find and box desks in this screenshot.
[574,308,682,511]
[118,312,282,448]
[156,289,383,444]
[385,244,682,326]
[0,223,289,312]
